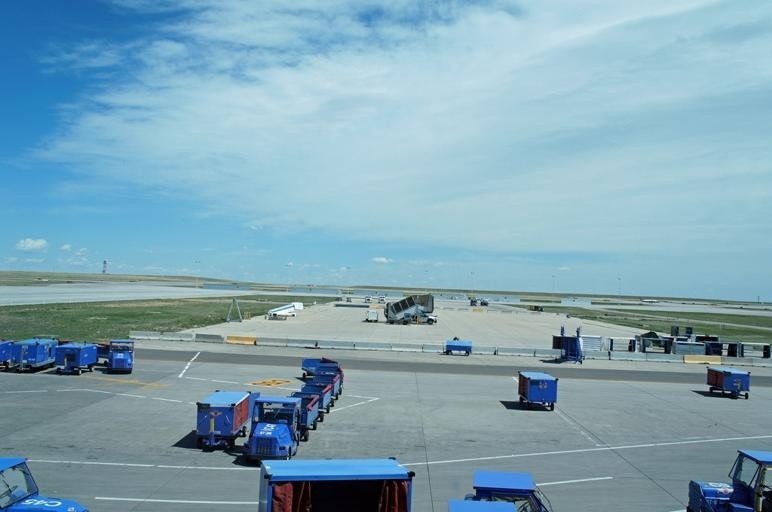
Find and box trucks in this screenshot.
[244,397,302,465]
[0,458,90,512]
[464,471,553,512]
[687,450,772,512]
[109,340,133,374]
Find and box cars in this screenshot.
[418,314,437,324]
